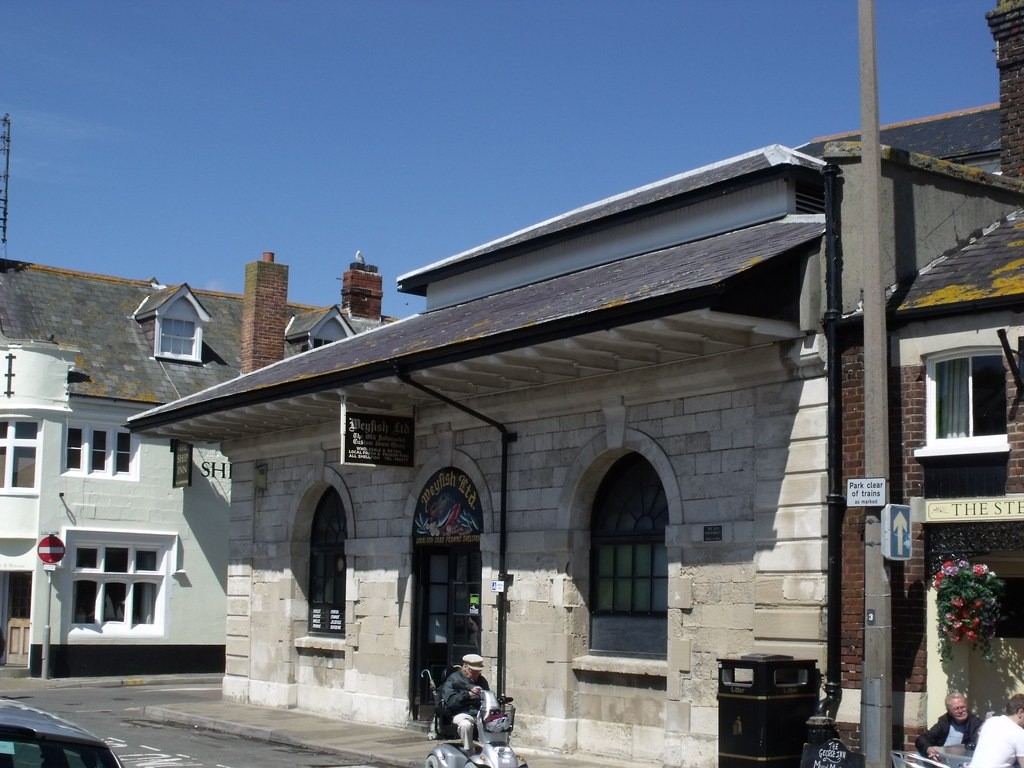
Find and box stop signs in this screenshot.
[37,535,65,564]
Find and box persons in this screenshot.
[969,693,1024,768]
[444,654,490,750]
[915,692,983,757]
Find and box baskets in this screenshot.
[480,705,516,733]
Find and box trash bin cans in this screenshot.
[717,653,819,768]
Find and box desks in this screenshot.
[935,744,977,768]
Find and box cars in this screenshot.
[0,698,125,768]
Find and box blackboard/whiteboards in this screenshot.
[799,738,866,768]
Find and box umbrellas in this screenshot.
[421,669,438,708]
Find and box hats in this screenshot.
[463,654,485,671]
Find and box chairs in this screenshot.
[420,665,480,741]
[891,750,949,768]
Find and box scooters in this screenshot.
[421,665,529,768]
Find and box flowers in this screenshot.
[932,559,1006,664]
